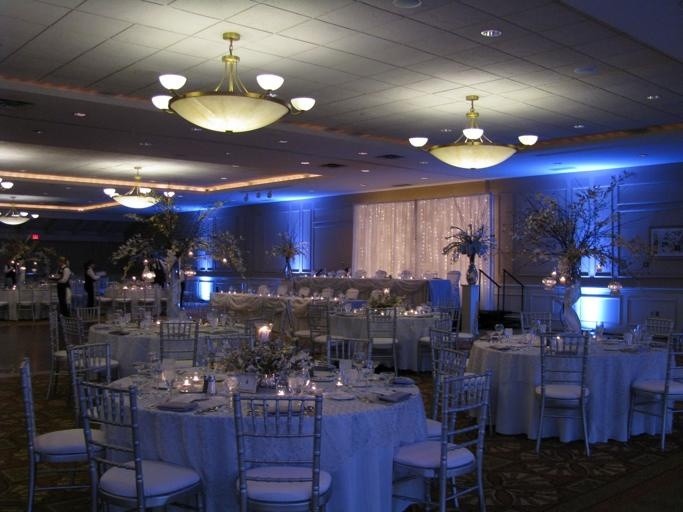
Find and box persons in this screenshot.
[82,260,100,313]
[3,257,15,288]
[49,255,72,318]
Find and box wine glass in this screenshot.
[488,319,652,348]
[102,307,234,337]
[151,353,373,396]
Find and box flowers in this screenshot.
[443,225,498,262]
[0,231,57,267]
[503,171,675,280]
[107,186,309,281]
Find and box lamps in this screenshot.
[0,197,39,225]
[407,95,538,170]
[103,166,174,209]
[151,32,316,134]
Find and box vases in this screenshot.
[465,253,478,285]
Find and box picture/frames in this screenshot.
[648,226,683,260]
